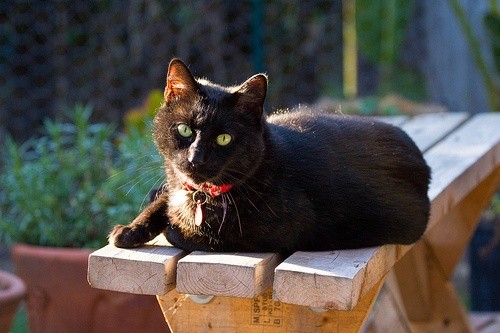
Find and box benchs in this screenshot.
[87,113,500,333]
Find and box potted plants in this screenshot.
[463,193,500,313]
[0,102,172,332]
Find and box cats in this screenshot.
[107,56,433,254]
